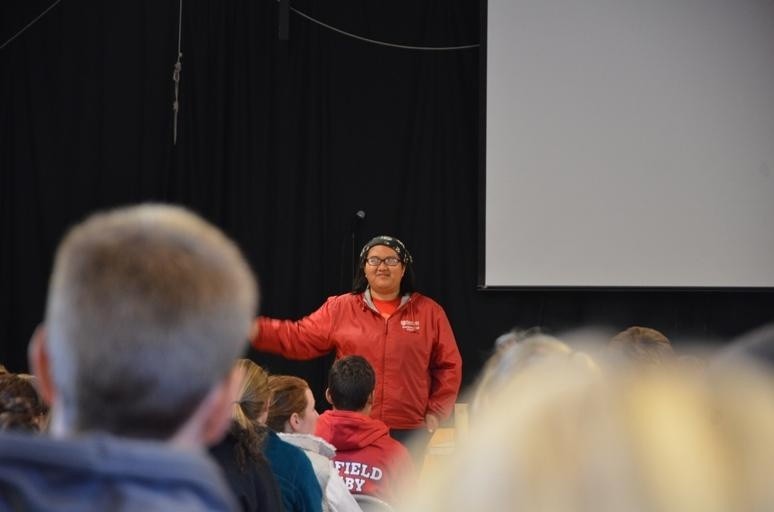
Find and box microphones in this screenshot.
[344,210,366,247]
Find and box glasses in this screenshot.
[366,256,402,265]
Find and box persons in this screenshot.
[0,202,683,511]
